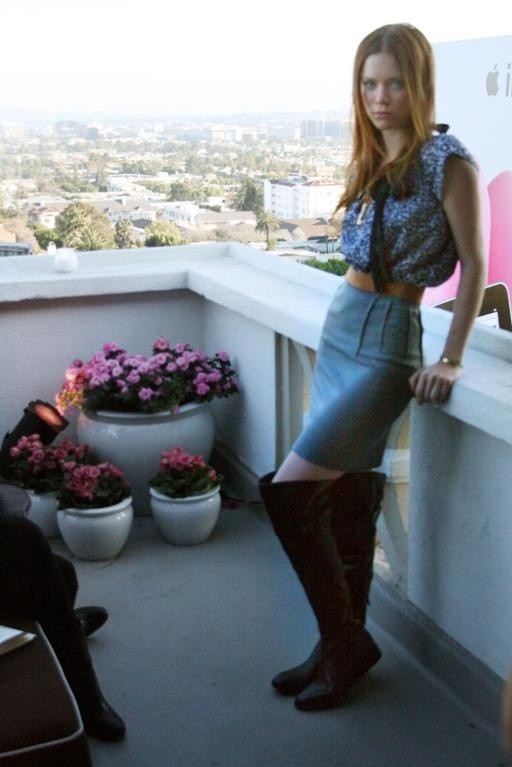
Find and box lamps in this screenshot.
[0,400,69,484]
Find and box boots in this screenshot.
[257,470,382,711]
[270,470,387,696]
[72,606,110,633]
[49,618,125,742]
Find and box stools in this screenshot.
[1,616,92,762]
[0,482,32,528]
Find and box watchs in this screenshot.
[440,355,460,369]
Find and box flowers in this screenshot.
[64,463,126,507]
[150,448,218,496]
[7,433,66,490]
[56,338,239,413]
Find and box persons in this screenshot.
[259,25,491,714]
[0,500,124,741]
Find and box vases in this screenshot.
[57,495,135,561]
[77,401,216,517]
[149,485,221,545]
[27,492,59,538]
